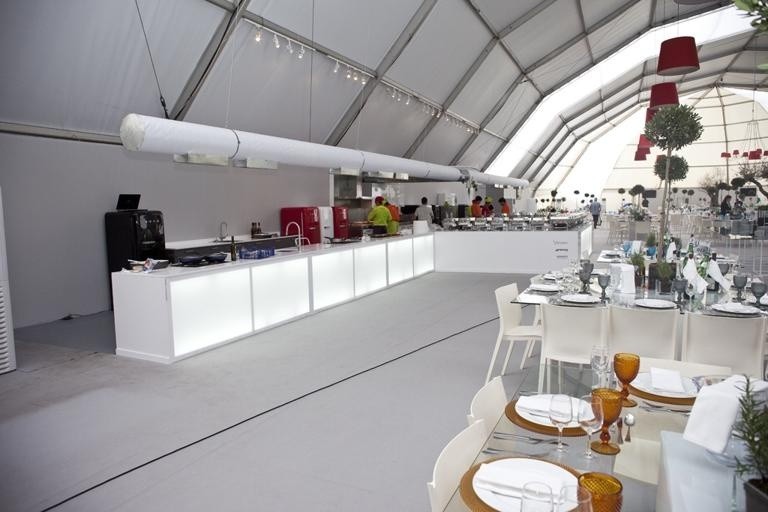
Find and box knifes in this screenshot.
[617,415,624,446]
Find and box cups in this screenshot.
[518,482,554,511]
[558,485,594,511]
[576,471,624,511]
[363,230,368,237]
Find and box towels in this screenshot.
[510,391,590,423]
[684,373,768,454]
[643,361,702,398]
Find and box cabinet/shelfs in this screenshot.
[161,224,372,266]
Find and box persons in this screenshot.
[641,197,649,207]
[414,197,436,224]
[471,195,482,217]
[590,197,602,230]
[498,197,510,217]
[382,198,401,235]
[481,196,494,217]
[719,194,733,219]
[367,196,393,234]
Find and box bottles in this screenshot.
[230,236,237,261]
[665,229,712,279]
[251,221,261,238]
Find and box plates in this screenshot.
[617,371,734,406]
[505,400,590,437]
[522,266,764,319]
[514,394,594,429]
[255,234,273,238]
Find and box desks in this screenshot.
[654,426,767,511]
[437,351,734,512]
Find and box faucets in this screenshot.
[216,221,228,241]
[285,221,302,252]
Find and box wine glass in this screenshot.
[590,342,612,386]
[614,352,640,408]
[621,241,630,258]
[577,395,605,460]
[589,386,624,455]
[548,393,574,457]
[577,257,768,313]
[648,247,657,260]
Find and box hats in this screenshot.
[375,196,384,202]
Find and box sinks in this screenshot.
[209,239,243,243]
[276,249,298,252]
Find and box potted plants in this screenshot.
[727,369,768,512]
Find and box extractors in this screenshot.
[332,167,408,183]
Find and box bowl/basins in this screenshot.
[177,253,228,266]
[149,260,170,270]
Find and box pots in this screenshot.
[323,236,346,243]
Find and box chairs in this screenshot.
[596,197,768,279]
[481,264,768,394]
[425,418,492,512]
[464,374,510,431]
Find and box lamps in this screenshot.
[241,14,482,140]
[713,30,767,162]
[632,14,700,162]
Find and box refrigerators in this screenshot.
[279,206,320,245]
[320,207,333,243]
[334,206,349,239]
[104,210,165,272]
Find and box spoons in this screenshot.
[624,411,635,444]
